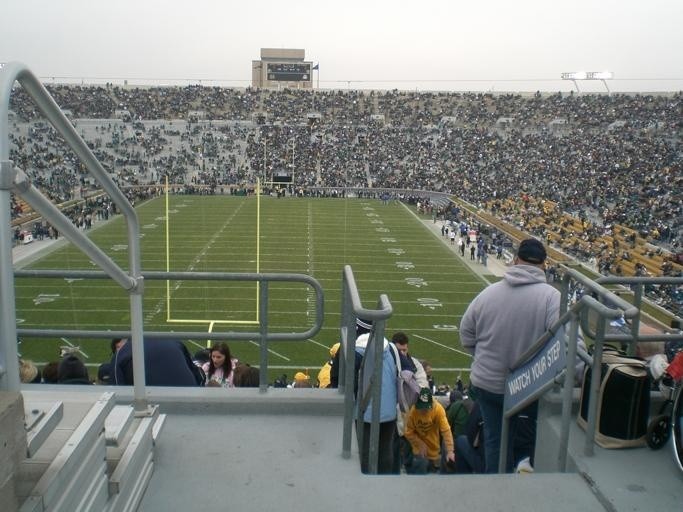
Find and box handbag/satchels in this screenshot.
[576,343,650,449]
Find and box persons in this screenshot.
[665,318,683,361]
[7,82,683,317]
[111,337,200,386]
[391,331,530,472]
[234,366,259,387]
[330,319,401,473]
[275,372,312,388]
[459,238,587,472]
[318,343,340,388]
[59,356,92,384]
[43,363,59,384]
[202,342,237,387]
[20,361,41,383]
[98,364,111,385]
[192,349,209,367]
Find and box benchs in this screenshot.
[10,87,682,221]
[494,212,682,323]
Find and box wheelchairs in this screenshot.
[646,351,683,470]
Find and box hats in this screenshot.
[416,388,432,409]
[520,239,546,264]
[294,373,310,380]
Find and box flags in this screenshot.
[313,64,318,70]
